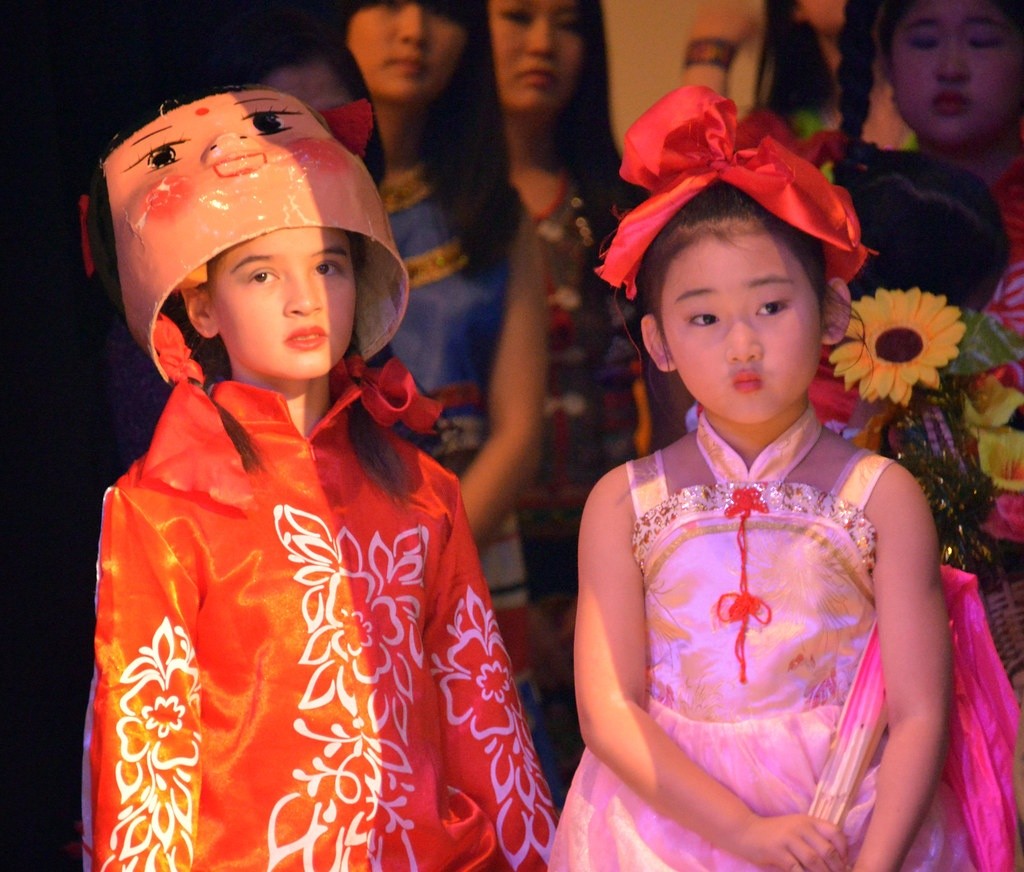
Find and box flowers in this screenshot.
[829,288,1024,574]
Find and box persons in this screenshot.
[483,1,698,800]
[78,164,561,872]
[101,2,442,478]
[70,81,413,388]
[754,0,920,185]
[541,171,974,871]
[679,0,771,104]
[321,0,564,812]
[804,128,1013,450]
[829,1,1022,417]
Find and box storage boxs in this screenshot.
[978,583,1020,700]
